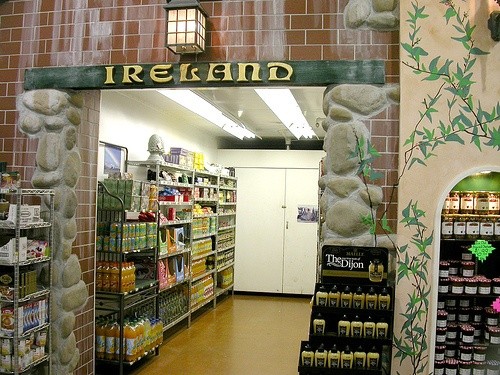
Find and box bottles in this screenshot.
[95,262,190,362]
[368,258,384,282]
[301,343,379,369]
[337,312,389,339]
[313,313,326,336]
[190,231,234,310]
[315,283,391,310]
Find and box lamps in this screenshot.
[156,88,320,141]
[163,0,209,54]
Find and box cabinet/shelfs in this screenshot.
[297,245,394,375]
[215,174,238,307]
[172,170,221,326]
[125,161,195,331]
[95,181,162,375]
[0,187,56,375]
[437,210,500,375]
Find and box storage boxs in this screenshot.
[0,170,49,337]
[97,147,204,290]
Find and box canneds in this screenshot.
[433,190,500,375]
[96,222,158,252]
